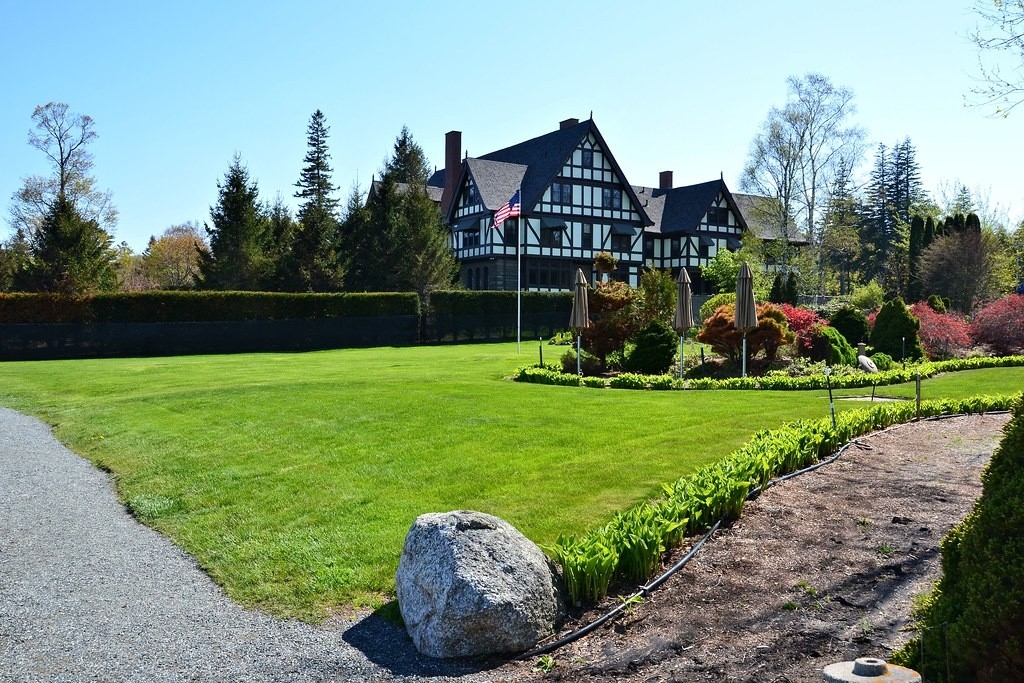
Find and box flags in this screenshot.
[491,190,521,228]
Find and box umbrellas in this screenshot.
[569,268,589,376]
[672,267,694,379]
[734,261,759,378]
